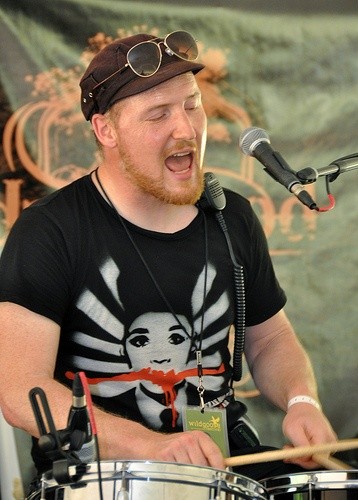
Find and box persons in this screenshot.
[0,31,337,500]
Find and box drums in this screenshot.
[259,470,358,500]
[24,461,269,500]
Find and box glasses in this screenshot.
[92,31,199,90]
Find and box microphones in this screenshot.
[239,127,316,210]
[68,372,97,463]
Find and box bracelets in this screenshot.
[289,395,319,407]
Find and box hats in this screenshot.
[79,34,205,120]
